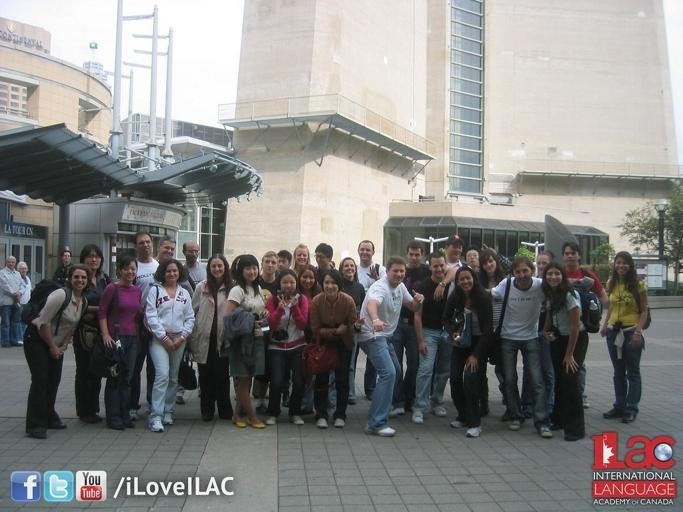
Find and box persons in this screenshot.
[1,230,651,442]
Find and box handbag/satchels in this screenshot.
[177,345,198,391]
[87,335,122,379]
[487,327,503,365]
[439,290,473,350]
[301,328,341,375]
[139,284,160,341]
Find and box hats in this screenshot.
[447,234,464,245]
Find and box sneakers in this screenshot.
[80,409,140,432]
[326,393,374,409]
[539,422,586,442]
[146,395,186,433]
[315,416,345,428]
[448,418,482,438]
[201,409,246,428]
[362,403,447,439]
[501,397,533,431]
[582,396,589,409]
[28,419,67,439]
[247,394,290,429]
[1,340,23,348]
[290,413,305,425]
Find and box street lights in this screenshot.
[653,202,670,262]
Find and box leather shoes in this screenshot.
[603,406,628,420]
[622,407,638,424]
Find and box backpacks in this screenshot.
[569,284,604,334]
[607,274,651,330]
[20,278,72,328]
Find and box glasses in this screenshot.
[183,250,200,252]
[314,254,328,258]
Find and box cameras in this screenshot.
[272,329,289,341]
[613,321,622,332]
[107,337,126,353]
